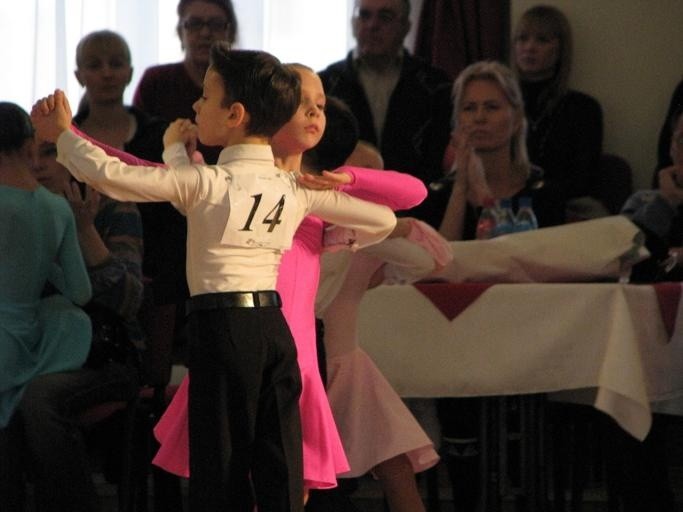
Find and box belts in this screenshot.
[186,292,279,312]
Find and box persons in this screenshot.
[127,0,238,169]
[0,31,683,512]
[313,0,455,233]
[505,0,607,227]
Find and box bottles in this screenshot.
[475,196,496,240]
[494,199,516,237]
[512,197,538,233]
